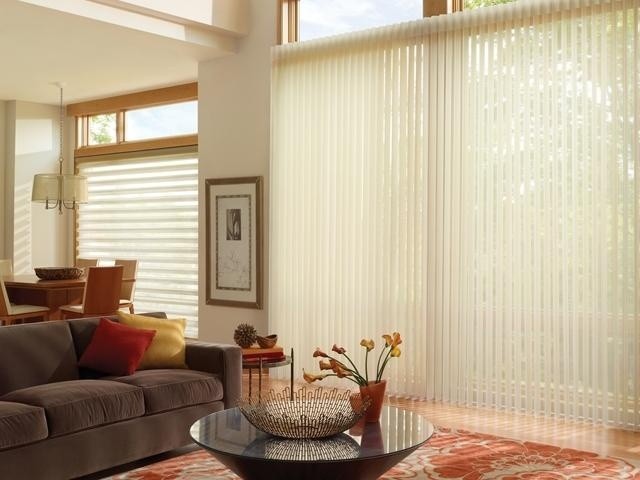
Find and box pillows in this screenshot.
[114,308,195,370]
[76,314,158,379]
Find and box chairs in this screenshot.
[0,257,139,319]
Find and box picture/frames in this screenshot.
[202,172,266,311]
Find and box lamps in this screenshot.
[28,85,92,216]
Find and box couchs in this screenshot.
[1,310,244,479]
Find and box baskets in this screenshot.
[33,265,86,281]
[255,333,279,347]
[232,382,372,440]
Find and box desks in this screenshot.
[188,398,436,480]
[233,346,298,406]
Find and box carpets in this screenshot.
[116,412,639,479]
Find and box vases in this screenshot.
[359,416,384,457]
[350,392,361,415]
[350,420,361,436]
[357,378,387,424]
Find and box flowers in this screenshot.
[300,329,405,390]
